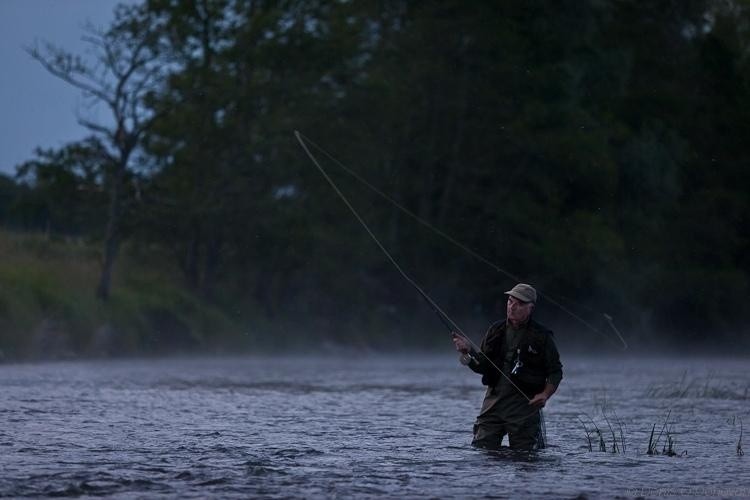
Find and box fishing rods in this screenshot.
[292,129,481,366]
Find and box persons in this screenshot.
[451,283,563,452]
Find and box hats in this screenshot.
[503,284,537,303]
